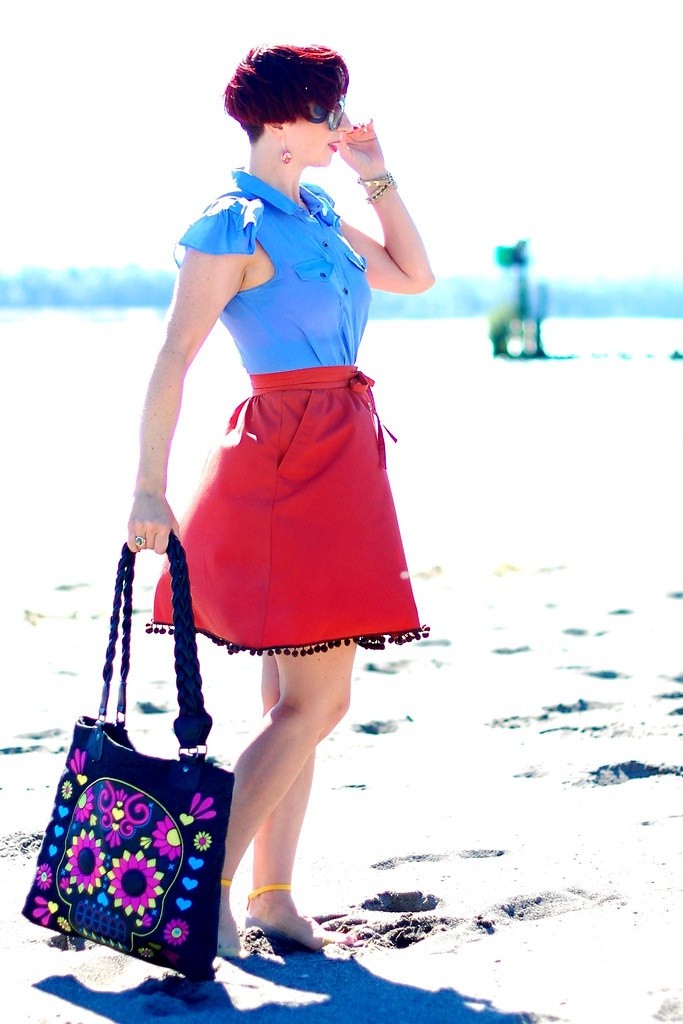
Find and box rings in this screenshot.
[135,536,147,547]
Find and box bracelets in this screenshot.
[355,171,398,205]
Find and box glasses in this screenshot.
[297,95,346,132]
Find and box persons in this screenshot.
[125,42,435,957]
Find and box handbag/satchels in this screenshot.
[21,532,235,984]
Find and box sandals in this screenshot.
[245,884,369,955]
[217,879,241,957]
